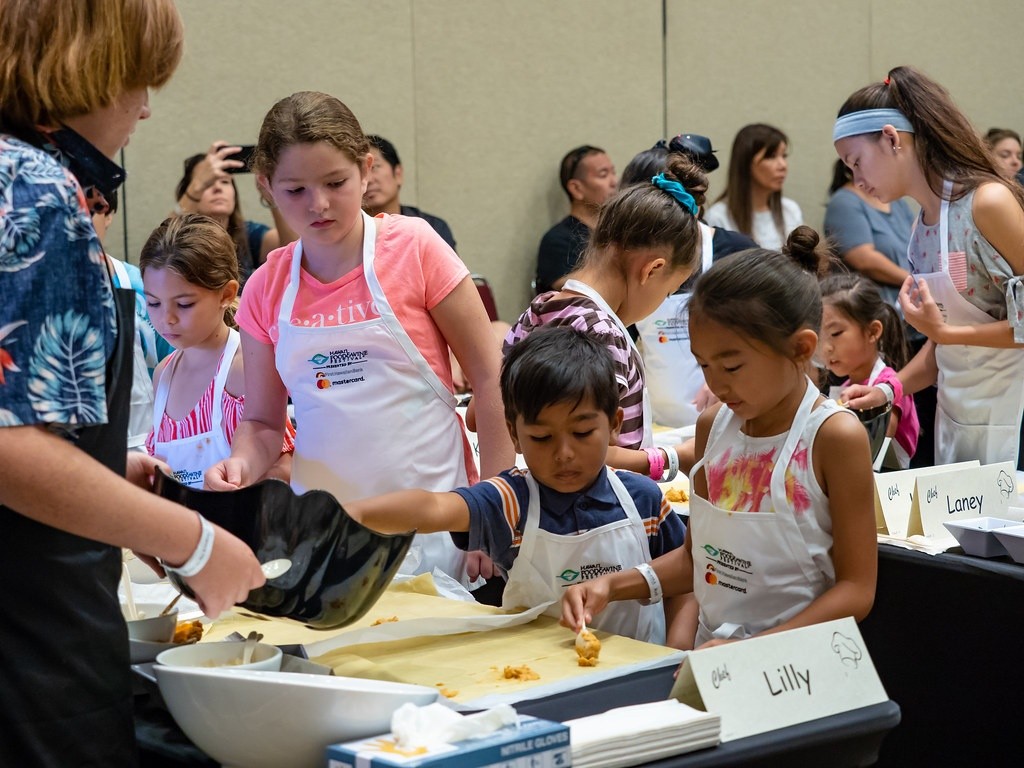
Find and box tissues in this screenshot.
[324,700,573,768]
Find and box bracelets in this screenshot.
[639,445,679,483]
[157,510,214,577]
[876,376,903,408]
[634,564,662,606]
[184,191,200,202]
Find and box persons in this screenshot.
[341,323,698,650]
[984,127,1024,179]
[0,0,265,768]
[465,133,709,483]
[834,66,1024,468]
[536,145,620,295]
[704,124,803,250]
[362,136,456,252]
[140,213,296,484]
[92,193,176,453]
[820,275,920,470]
[618,133,826,430]
[822,158,927,342]
[560,226,877,676]
[204,91,515,608]
[173,141,279,298]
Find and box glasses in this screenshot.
[568,145,605,178]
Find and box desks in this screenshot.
[856,538,1023,768]
[110,553,901,768]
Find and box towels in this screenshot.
[560,697,722,768]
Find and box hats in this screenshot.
[670,135,721,173]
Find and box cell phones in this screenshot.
[217,146,258,174]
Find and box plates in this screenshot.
[129,631,332,683]
[649,469,692,517]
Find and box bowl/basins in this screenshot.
[152,663,440,768]
[118,600,203,663]
[170,608,211,626]
[942,517,1024,564]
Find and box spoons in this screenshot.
[260,558,292,579]
[154,640,283,674]
